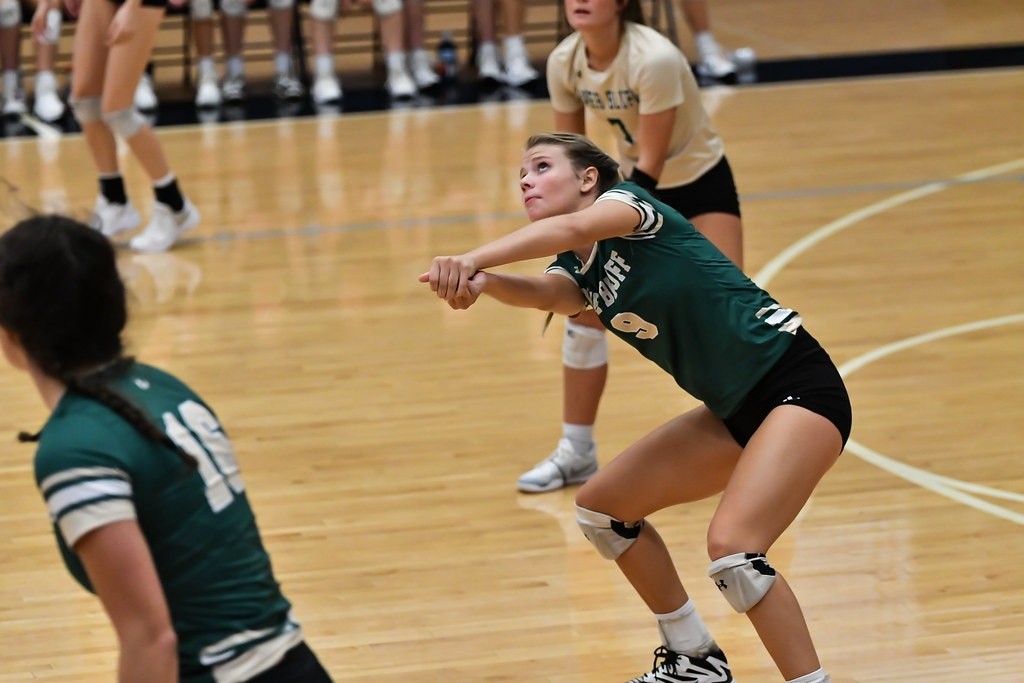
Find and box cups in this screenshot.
[734,47,756,83]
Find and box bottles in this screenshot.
[437,31,458,78]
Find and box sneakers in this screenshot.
[505,57,540,87]
[130,195,202,252]
[35,82,65,120]
[312,74,341,104]
[274,72,302,97]
[410,62,441,89]
[627,645,733,683]
[519,496,589,534]
[388,72,417,97]
[223,72,246,100]
[698,57,736,78]
[518,438,597,493]
[479,58,502,80]
[132,70,159,110]
[195,70,221,107]
[1,94,28,115]
[86,192,140,237]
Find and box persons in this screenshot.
[169,0,249,105]
[0,214,335,683]
[372,0,440,98]
[69,0,202,254]
[419,133,852,683]
[677,0,738,78]
[267,0,343,103]
[516,0,743,494]
[0,0,65,120]
[469,0,538,86]
